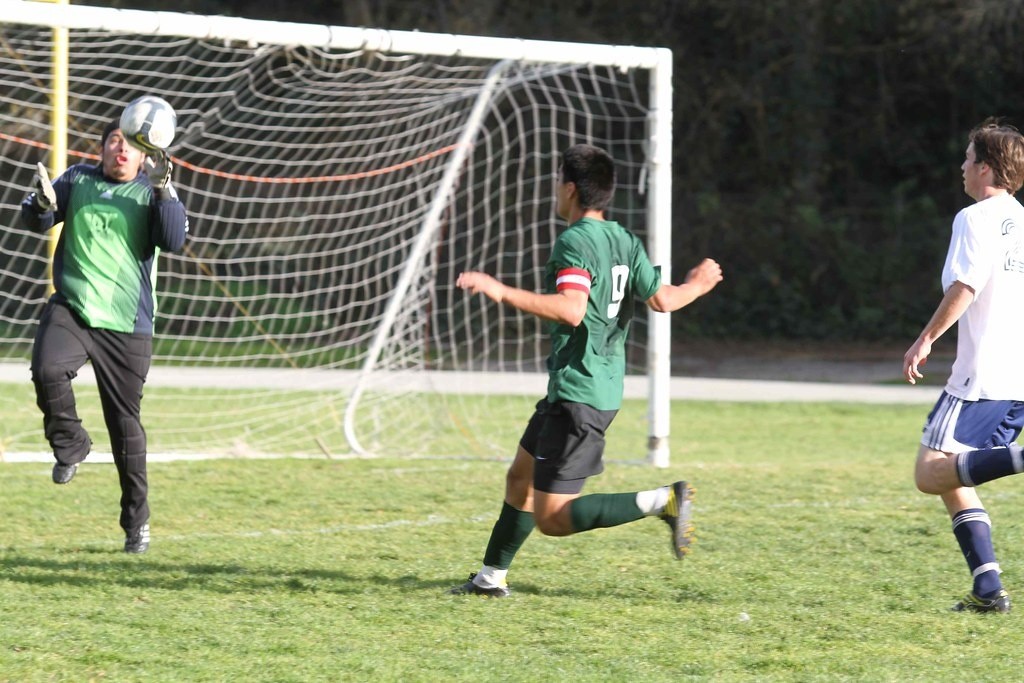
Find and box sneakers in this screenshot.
[52,439,93,483]
[660,481,696,560]
[124,519,149,553]
[451,572,510,597]
[950,588,1010,613]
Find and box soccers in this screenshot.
[118,95,180,152]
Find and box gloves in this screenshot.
[144,150,173,188]
[35,162,57,211]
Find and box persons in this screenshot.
[21,116,188,553]
[455,143,723,597]
[902,124,1024,613]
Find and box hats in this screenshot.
[100,116,120,147]
